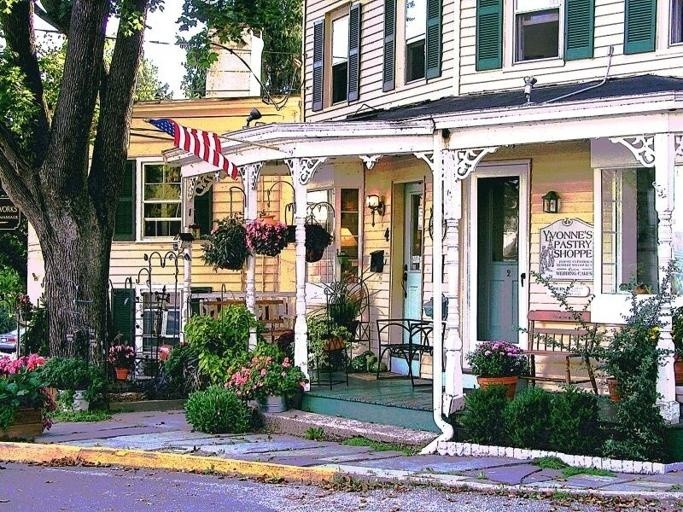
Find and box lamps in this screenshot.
[340,227,357,246]
[247,108,261,126]
[523,76,537,102]
[542,191,559,213]
[366,194,385,227]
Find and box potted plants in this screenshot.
[304,224,333,262]
[671,307,683,387]
[320,325,351,350]
[202,215,251,270]
[331,300,359,342]
[618,261,655,294]
[511,259,680,402]
[47,355,104,412]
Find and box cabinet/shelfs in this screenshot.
[316,286,348,390]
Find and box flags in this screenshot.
[145,118,239,179]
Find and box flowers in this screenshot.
[107,344,135,367]
[0,354,47,405]
[466,337,530,377]
[224,355,308,399]
[245,218,289,256]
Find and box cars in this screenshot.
[0,330,18,353]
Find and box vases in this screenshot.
[7,404,44,439]
[114,366,128,380]
[476,376,518,402]
[263,394,287,413]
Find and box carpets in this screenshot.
[306,365,432,411]
[346,371,402,381]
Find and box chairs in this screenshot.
[377,319,446,387]
[522,310,599,395]
